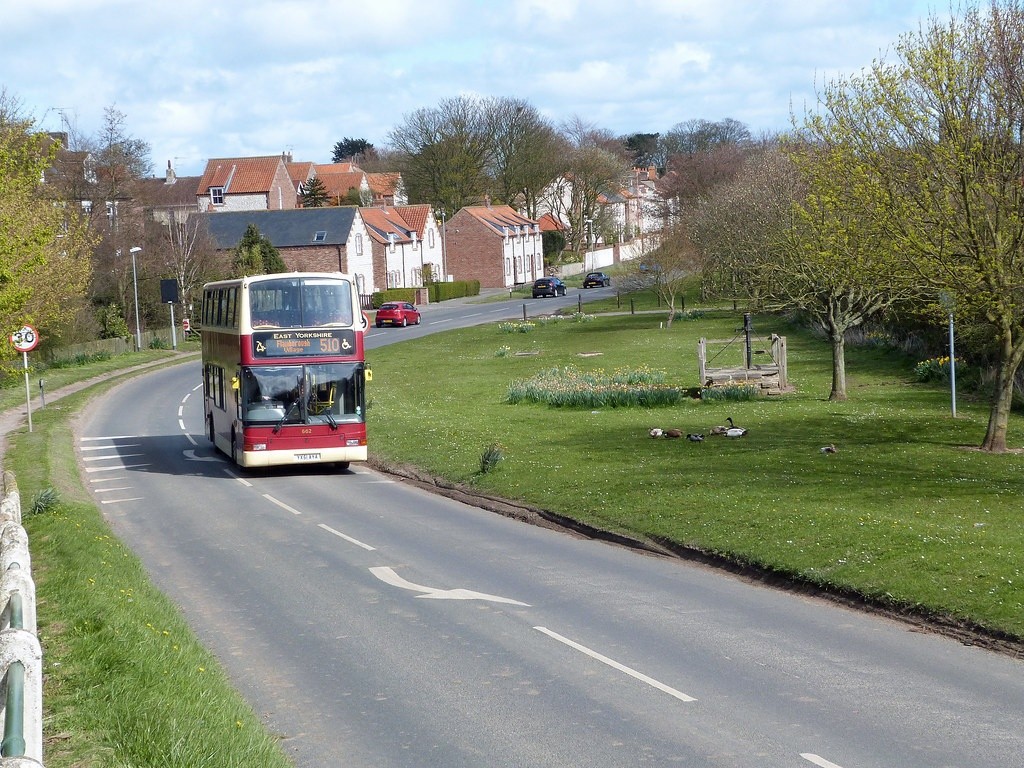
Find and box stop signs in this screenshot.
[182,321,189,330]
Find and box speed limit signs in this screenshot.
[9,324,38,352]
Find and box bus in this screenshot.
[200,271,373,471]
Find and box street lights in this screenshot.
[130,247,142,351]
[442,213,446,282]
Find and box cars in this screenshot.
[584,272,610,289]
[639,257,664,273]
[376,301,422,327]
[533,276,568,299]
